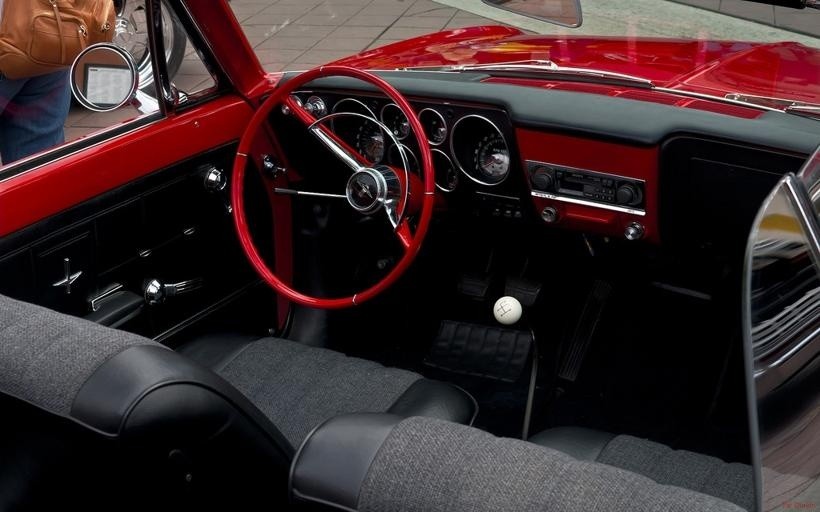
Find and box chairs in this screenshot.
[288,412,752,511]
[1,293,479,511]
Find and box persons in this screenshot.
[0,68,73,166]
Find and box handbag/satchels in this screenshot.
[0,1,116,80]
